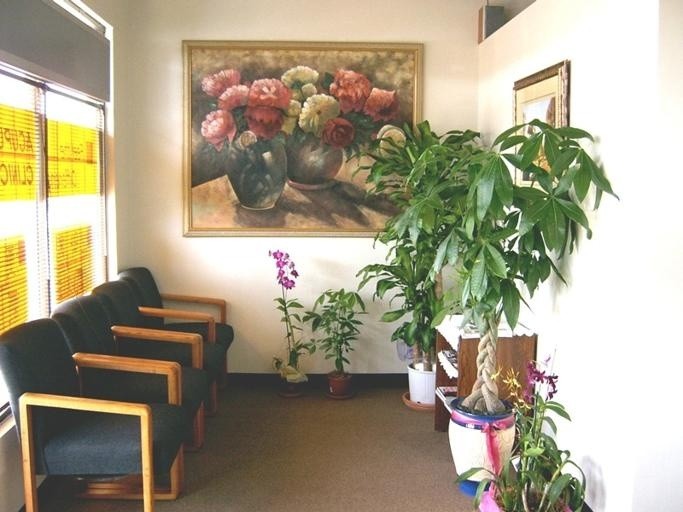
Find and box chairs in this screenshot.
[1,318,191,512]
[51,294,212,485]
[91,281,226,452]
[117,267,233,414]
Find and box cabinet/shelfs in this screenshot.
[434,315,536,432]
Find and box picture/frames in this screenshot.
[181,40,424,233]
[512,59,570,193]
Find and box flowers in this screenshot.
[451,346,591,510]
[268,248,313,385]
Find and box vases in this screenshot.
[274,375,305,398]
[474,481,577,511]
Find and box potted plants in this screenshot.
[302,286,370,398]
[346,119,460,409]
[433,123,620,495]
